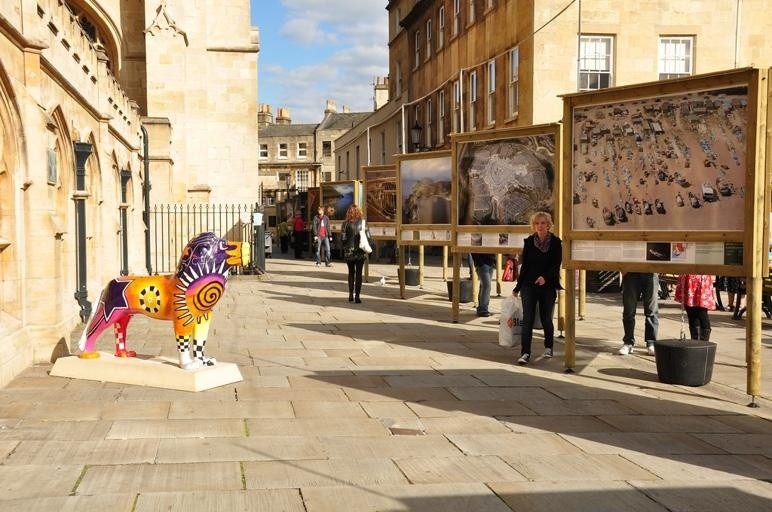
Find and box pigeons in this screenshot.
[380,276,386,286]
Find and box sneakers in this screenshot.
[544,348,553,359]
[316,263,332,267]
[617,343,633,356]
[517,352,529,364]
[646,344,654,355]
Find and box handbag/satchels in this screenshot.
[358,219,372,254]
[499,296,524,347]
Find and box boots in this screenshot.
[349,295,361,304]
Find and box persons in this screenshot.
[470,253,499,317]
[340,204,373,304]
[724,274,743,313]
[278,217,289,254]
[311,205,334,267]
[466,252,477,281]
[291,210,308,260]
[616,269,663,357]
[673,271,718,342]
[511,212,564,365]
